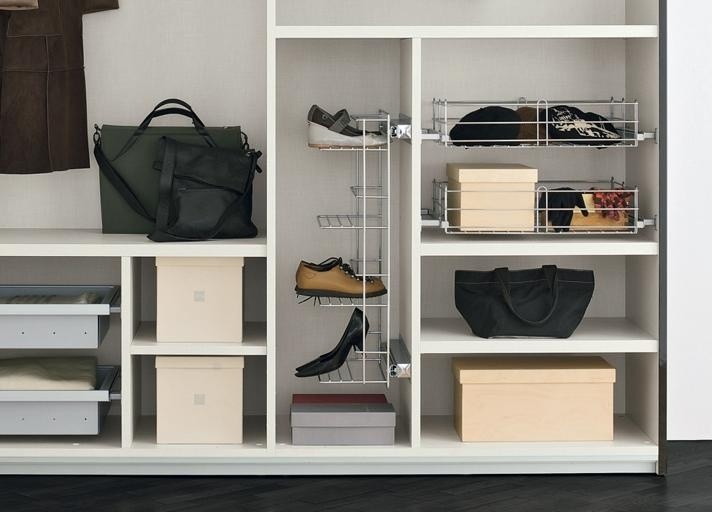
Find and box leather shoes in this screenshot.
[305,106,392,148]
[295,257,387,297]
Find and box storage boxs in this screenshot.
[152,353,246,445]
[287,392,398,447]
[446,356,618,444]
[444,159,540,235]
[153,254,246,346]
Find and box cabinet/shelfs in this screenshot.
[0,226,267,447]
[422,95,657,242]
[301,108,414,387]
[413,224,660,360]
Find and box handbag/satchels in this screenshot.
[93,99,262,241]
[454,264,595,337]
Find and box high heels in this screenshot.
[294,306,369,377]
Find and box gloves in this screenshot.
[538,187,589,232]
